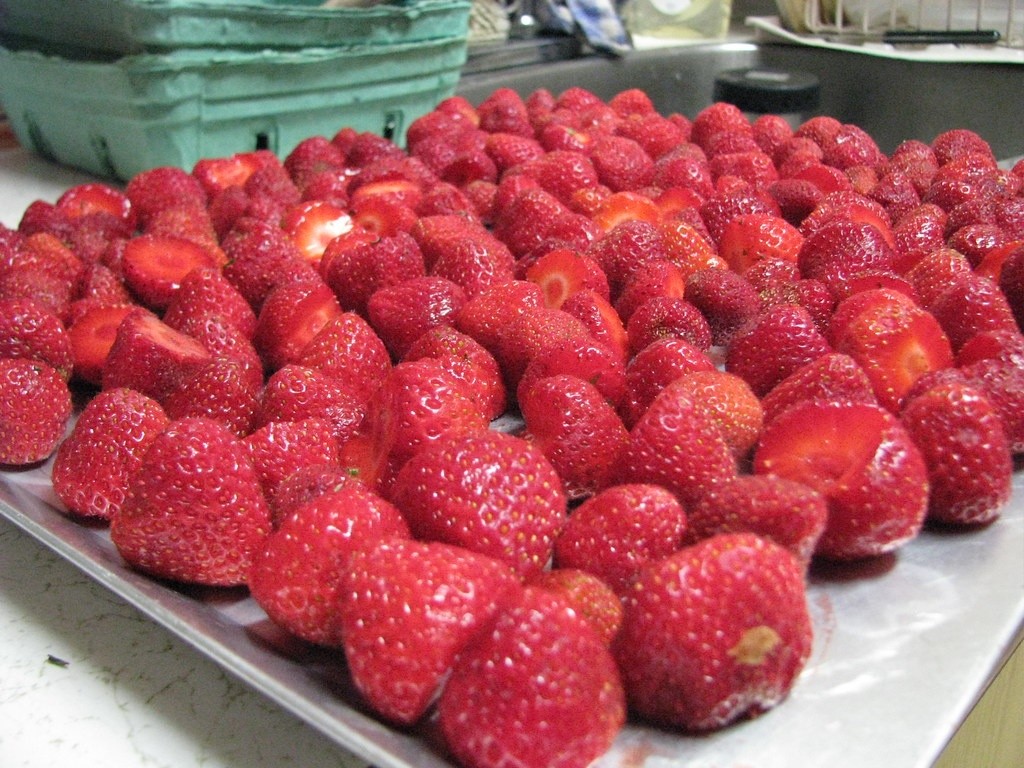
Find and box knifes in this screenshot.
[799,30,999,42]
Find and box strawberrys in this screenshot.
[0,86,1024,768]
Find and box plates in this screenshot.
[0,410,1024,768]
